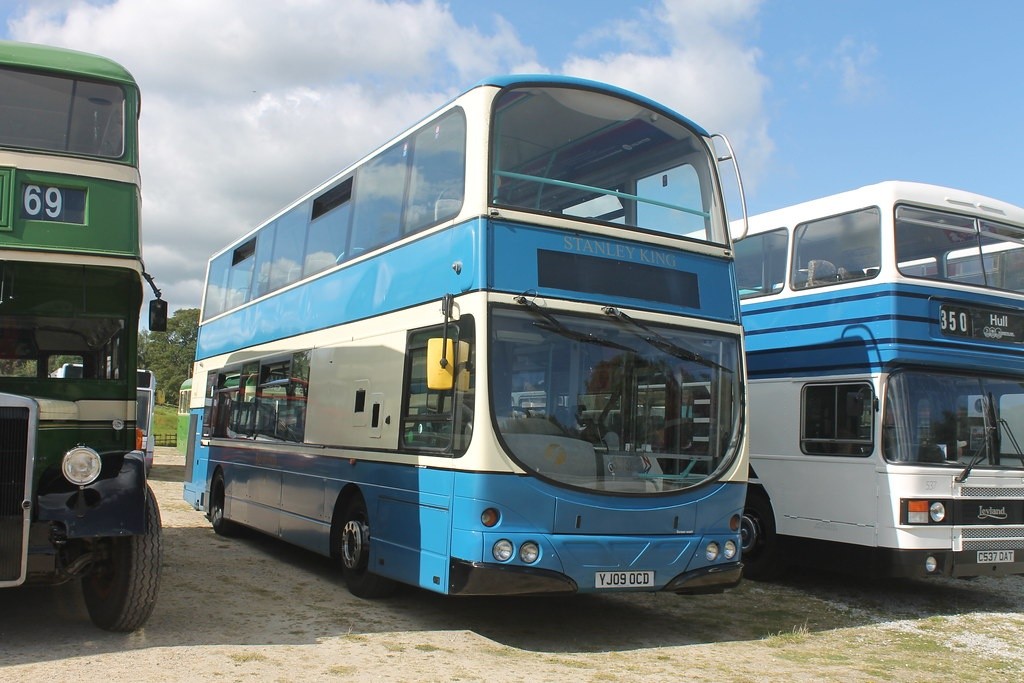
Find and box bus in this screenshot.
[180,71,751,597]
[0,35,168,633]
[46,362,165,480]
[680,179,1024,580]
[734,235,1024,470]
[177,373,193,457]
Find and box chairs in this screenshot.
[257,261,302,299]
[220,294,253,315]
[903,443,946,463]
[867,269,879,275]
[435,187,465,221]
[405,205,435,234]
[838,267,866,282]
[806,260,837,288]
[303,251,337,275]
